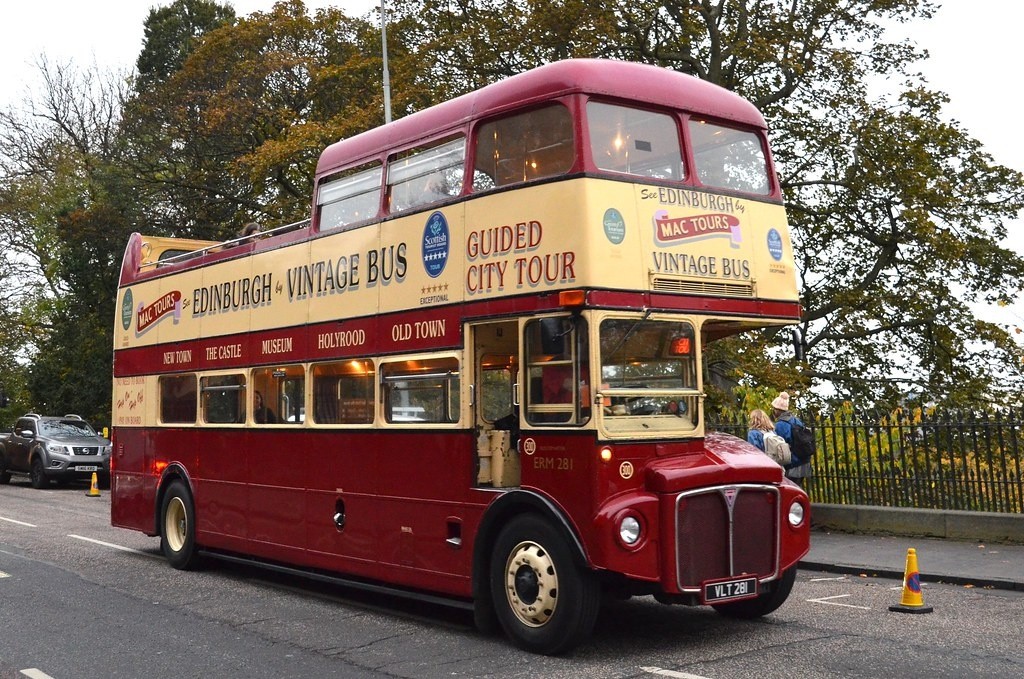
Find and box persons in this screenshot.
[747,409,786,477]
[207,382,234,423]
[239,390,276,424]
[542,338,590,418]
[769,392,819,532]
[223,222,258,249]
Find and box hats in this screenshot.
[772,392,790,411]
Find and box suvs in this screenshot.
[0,412,112,489]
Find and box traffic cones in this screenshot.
[888,547,933,614]
[85,472,102,497]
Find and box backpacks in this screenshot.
[758,429,791,465]
[780,417,815,459]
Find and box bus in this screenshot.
[104,56,815,660]
